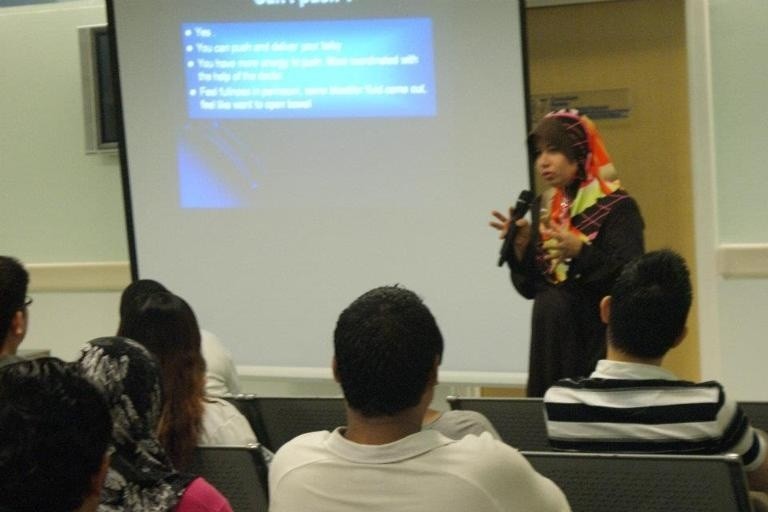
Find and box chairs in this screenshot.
[739,401,767,434]
[446,395,548,450]
[521,450,749,510]
[194,446,269,510]
[224,395,348,451]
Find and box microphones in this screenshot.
[497,190,534,266]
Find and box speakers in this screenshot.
[78,22,119,154]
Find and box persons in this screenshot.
[540,242,768,512]
[489,108,646,398]
[0,354,118,511]
[0,253,34,363]
[69,334,233,512]
[116,288,275,465]
[120,277,243,395]
[266,281,572,512]
